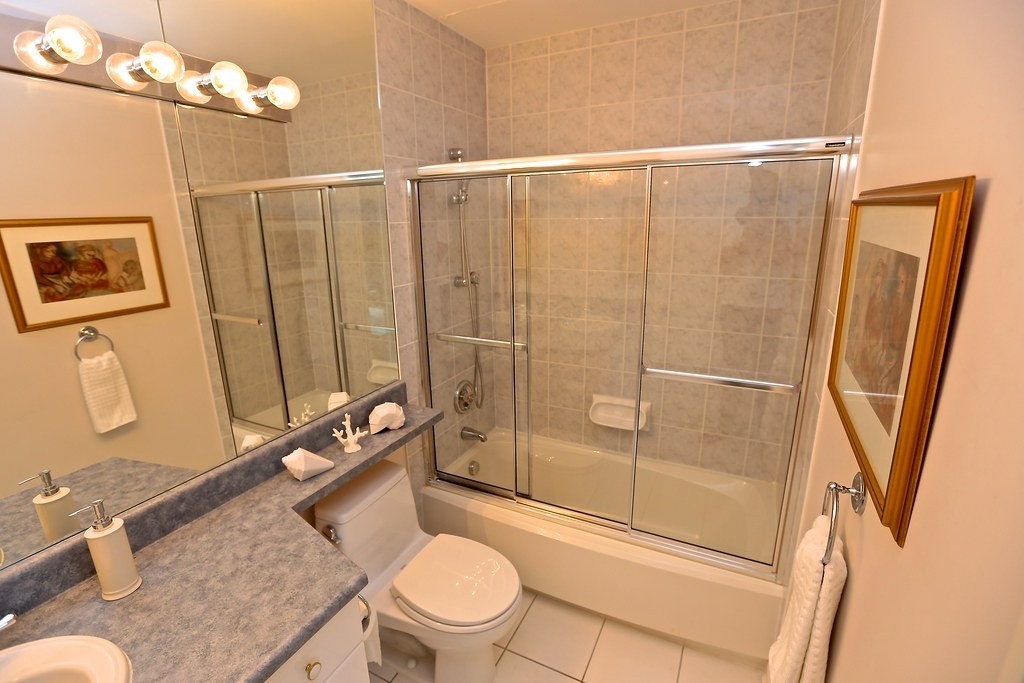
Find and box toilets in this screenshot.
[308,455,523,683]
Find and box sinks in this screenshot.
[0,633,133,683]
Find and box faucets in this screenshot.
[461,427,488,443]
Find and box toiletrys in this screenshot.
[67,498,143,604]
[18,464,84,547]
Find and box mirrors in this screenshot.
[0,71,403,571]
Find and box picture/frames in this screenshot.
[0,216,171,334]
[825,175,978,549]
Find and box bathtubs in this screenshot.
[423,422,792,665]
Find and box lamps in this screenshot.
[259,76,301,111]
[13,30,69,75]
[105,52,150,92]
[233,83,266,115]
[42,13,103,66]
[176,70,214,104]
[135,40,186,84]
[205,61,249,99]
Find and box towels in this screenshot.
[757,514,849,683]
[78,351,138,437]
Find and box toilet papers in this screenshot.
[355,599,385,669]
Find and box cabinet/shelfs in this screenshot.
[263,593,370,683]
